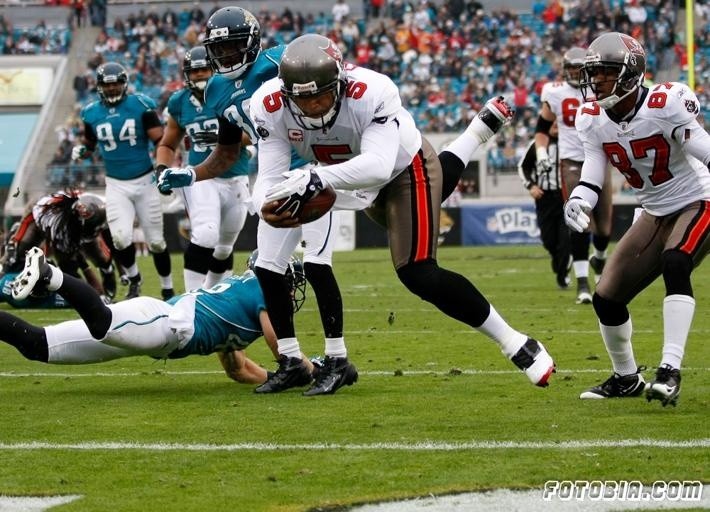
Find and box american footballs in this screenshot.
[278,185,337,224]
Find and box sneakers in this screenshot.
[10,244,45,302]
[514,335,558,388]
[469,96,514,142]
[100,265,140,302]
[70,143,89,164]
[252,354,360,397]
[577,366,684,406]
[553,255,606,302]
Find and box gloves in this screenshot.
[533,148,554,171]
[154,162,194,195]
[562,192,595,234]
[263,168,328,220]
[190,129,221,147]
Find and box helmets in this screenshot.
[564,32,650,111]
[183,6,263,89]
[278,32,344,133]
[94,61,129,106]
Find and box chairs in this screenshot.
[0,0,710,197]
